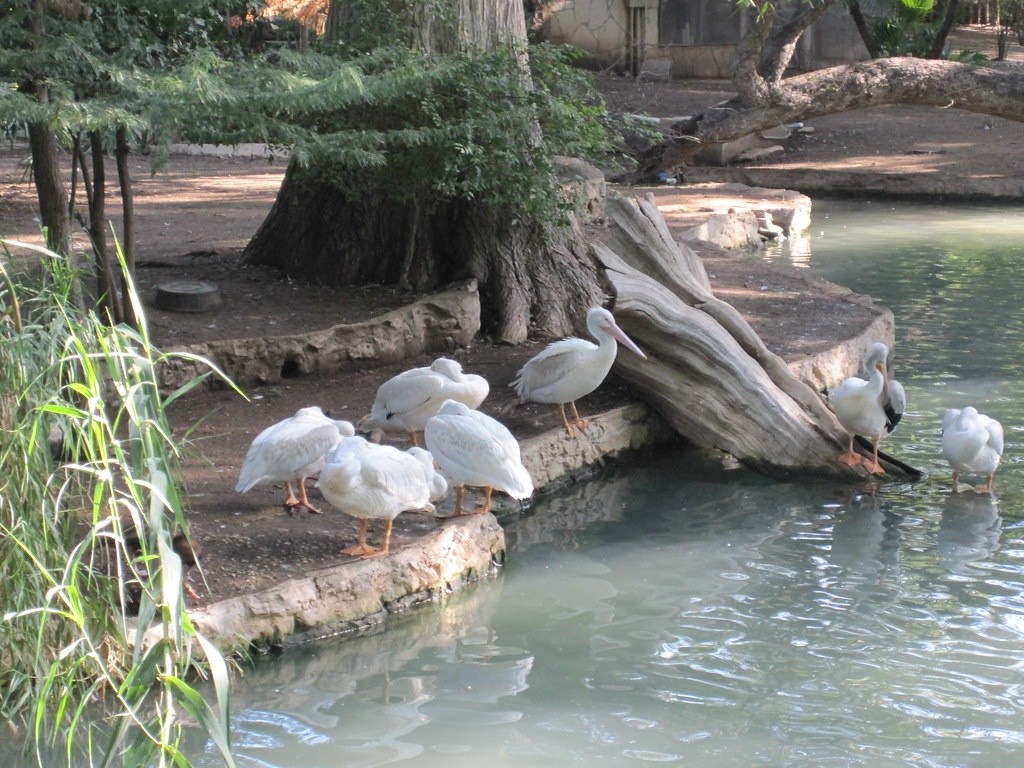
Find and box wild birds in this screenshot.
[507,306,647,438]
[941,407,1003,495]
[358,357,490,446]
[831,342,905,474]
[424,398,534,519]
[234,406,449,559]
